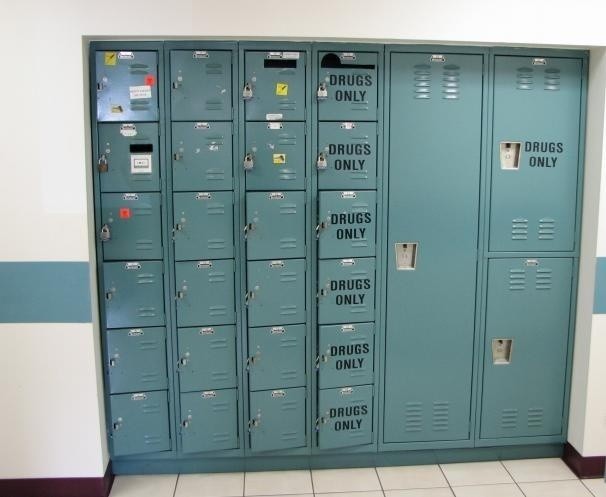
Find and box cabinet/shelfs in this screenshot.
[380,36,489,469]
[477,256,583,462]
[490,38,589,255]
[90,40,378,472]
